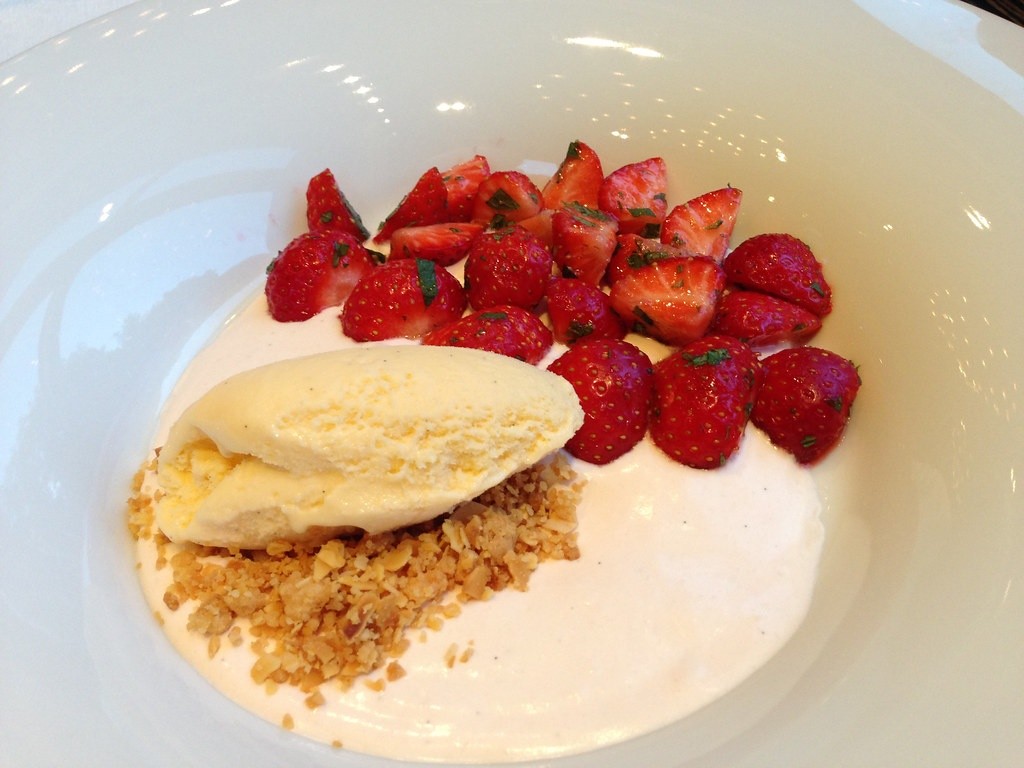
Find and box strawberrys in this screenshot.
[263,138,863,467]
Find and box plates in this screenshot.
[0,3,1021,768]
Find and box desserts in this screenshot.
[150,345,584,549]
